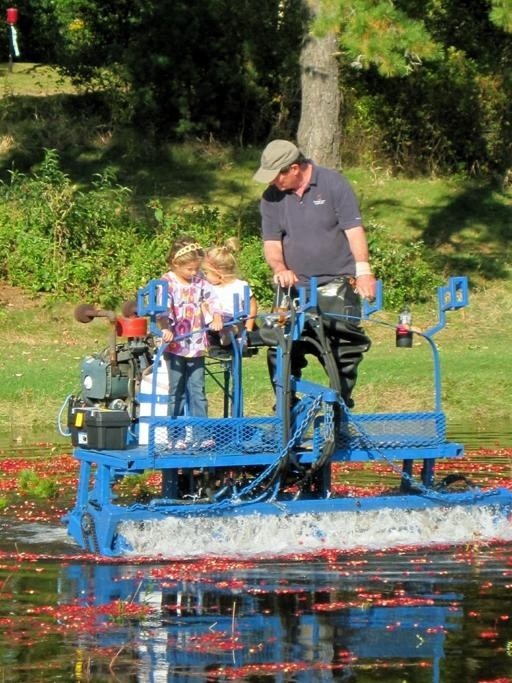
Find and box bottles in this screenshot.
[399,308,412,336]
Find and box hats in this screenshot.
[253,140,300,184]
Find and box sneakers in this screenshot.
[167,441,185,450]
[192,439,214,450]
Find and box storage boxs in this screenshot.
[67,406,130,451]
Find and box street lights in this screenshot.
[6,6,17,70]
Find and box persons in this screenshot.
[252,138,377,449]
[201,235,258,347]
[153,236,224,453]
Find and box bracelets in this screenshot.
[355,261,374,279]
